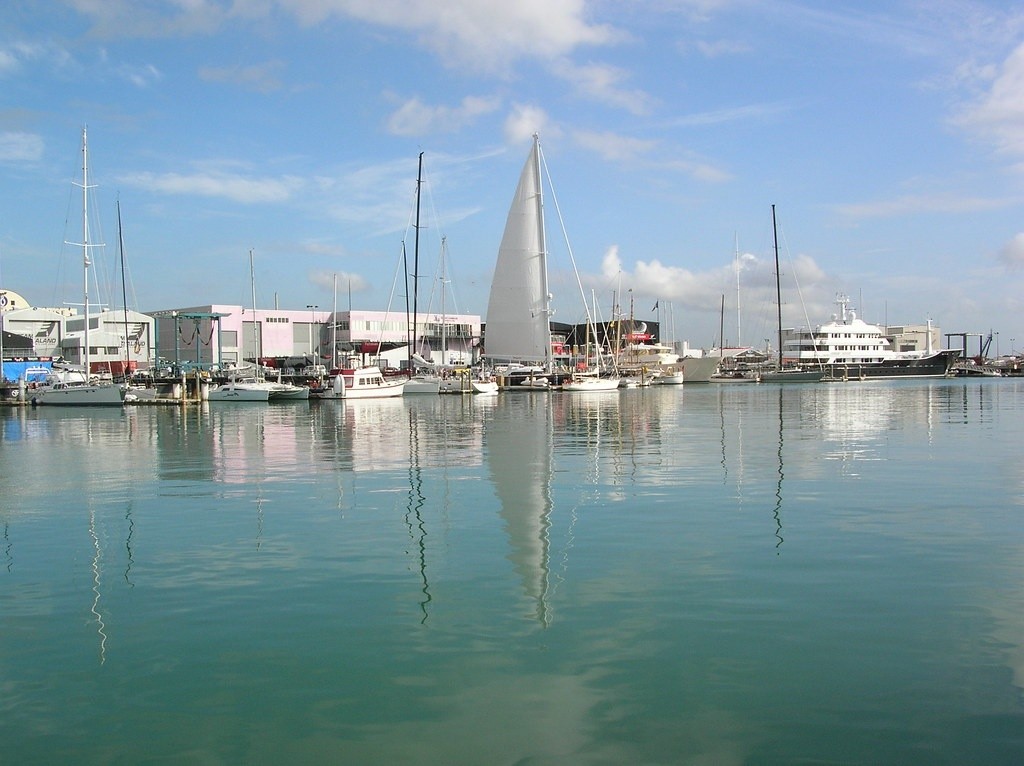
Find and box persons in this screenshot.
[303,380,310,389]
[57,355,64,361]
[167,365,173,377]
[125,365,134,378]
[312,380,318,389]
[443,368,490,384]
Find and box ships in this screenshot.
[762,294,965,380]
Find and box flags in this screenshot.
[652,301,658,311]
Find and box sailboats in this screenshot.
[15,120,835,409]
